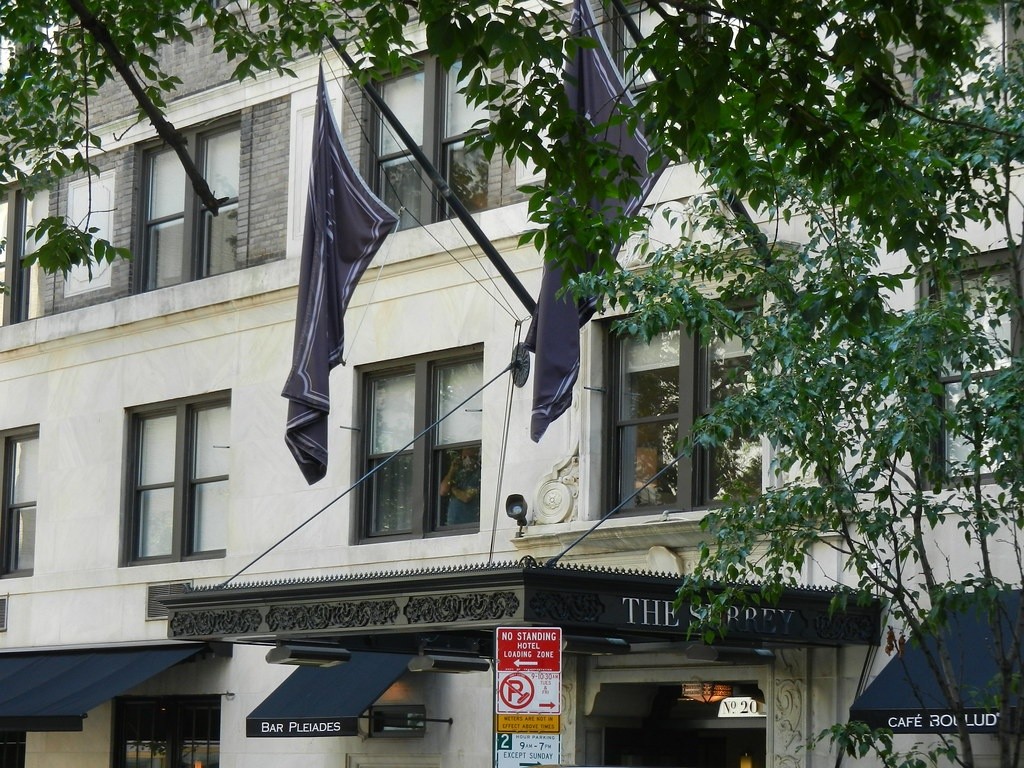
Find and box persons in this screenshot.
[439,447,481,525]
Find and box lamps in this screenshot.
[407,640,489,672]
[682,682,731,703]
[506,493,530,536]
[266,645,351,668]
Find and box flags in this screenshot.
[522,0,674,444]
[280,60,400,485]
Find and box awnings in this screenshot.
[0,643,205,732]
[245,651,417,737]
[849,589,1024,735]
